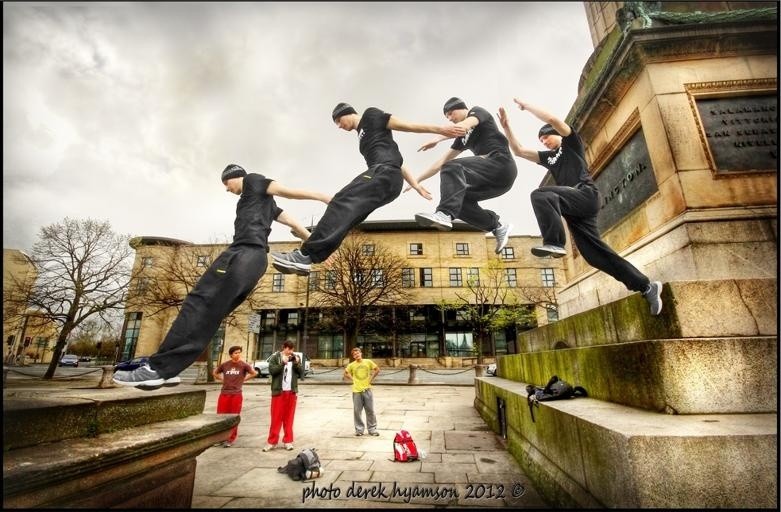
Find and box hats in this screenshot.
[332,102,357,121]
[444,97,467,113]
[538,123,561,137]
[221,164,245,180]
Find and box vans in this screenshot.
[252,351,312,377]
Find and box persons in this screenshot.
[404,98,519,256]
[212,345,257,448]
[261,341,304,452]
[342,347,381,435]
[496,97,664,317]
[268,103,468,273]
[111,165,332,388]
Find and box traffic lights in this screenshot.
[7,335,13,346]
[95,342,101,349]
[24,337,30,347]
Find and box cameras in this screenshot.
[288,355,295,362]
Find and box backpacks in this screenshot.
[526,375,587,402]
[394,430,418,462]
[283,448,320,480]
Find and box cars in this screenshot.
[60,354,79,368]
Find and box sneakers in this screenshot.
[415,211,452,232]
[263,444,277,452]
[270,249,313,277]
[284,442,293,450]
[643,281,663,315]
[215,441,231,447]
[113,366,182,390]
[532,245,565,258]
[492,218,513,254]
[356,429,379,436]
